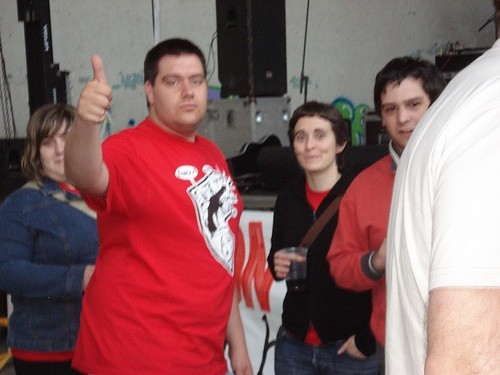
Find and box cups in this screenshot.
[285,248,309,279]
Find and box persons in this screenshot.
[266,101,384,375]
[64,38,253,375]
[327,56,449,348]
[0,103,101,375]
[384,38,500,375]
